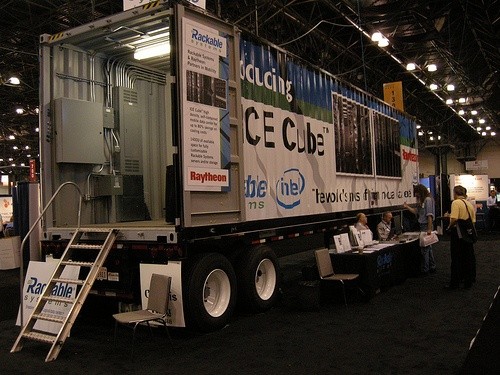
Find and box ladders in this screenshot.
[10,227,118,363]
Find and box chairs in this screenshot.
[314,249,359,309]
[113,273,172,362]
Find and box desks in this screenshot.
[326,232,424,294]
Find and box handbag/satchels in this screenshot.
[421,232,439,247]
[456,217,478,244]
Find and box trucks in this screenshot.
[9,0,421,364]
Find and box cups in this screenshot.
[358,245,364,254]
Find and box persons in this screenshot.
[403,184,436,274]
[444,185,477,290]
[377,211,396,240]
[356,213,373,239]
[487,190,498,232]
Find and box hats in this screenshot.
[490,186,495,192]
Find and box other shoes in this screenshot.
[443,285,463,291]
